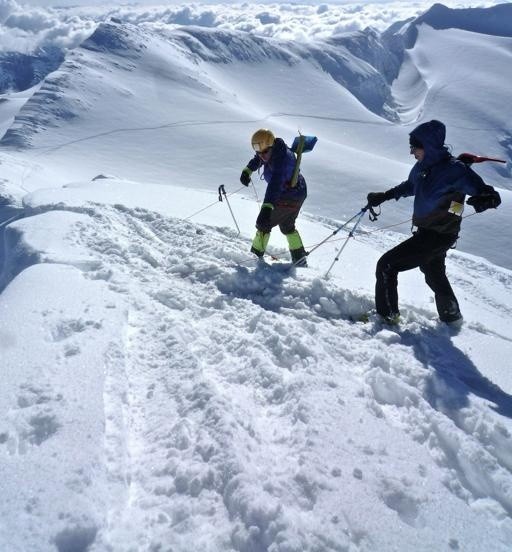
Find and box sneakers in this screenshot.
[427,310,464,328]
[359,308,402,326]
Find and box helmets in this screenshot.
[251,128,276,153]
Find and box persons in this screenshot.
[351,119,501,327]
[241,129,309,267]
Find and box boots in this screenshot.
[289,245,308,268]
[250,246,265,258]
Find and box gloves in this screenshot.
[465,194,497,214]
[240,169,252,188]
[366,191,387,206]
